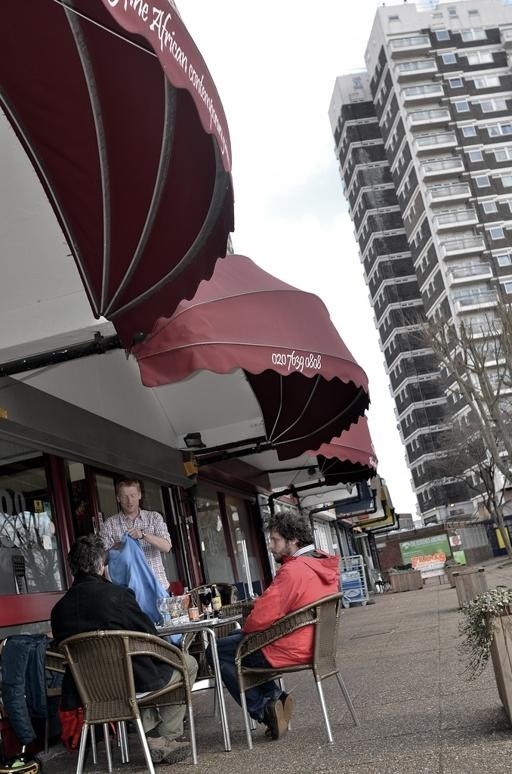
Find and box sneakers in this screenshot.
[263,700,286,739]
[147,737,191,764]
[263,693,294,736]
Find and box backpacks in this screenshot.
[59,707,117,751]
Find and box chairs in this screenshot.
[58,631,198,774]
[186,583,238,613]
[183,604,255,720]
[0,637,121,753]
[234,592,361,751]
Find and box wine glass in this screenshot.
[252,593,258,599]
[156,595,185,628]
[198,588,212,621]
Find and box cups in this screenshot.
[189,607,199,622]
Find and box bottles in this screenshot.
[211,584,222,619]
[182,587,192,622]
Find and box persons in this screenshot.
[207,512,339,741]
[97,479,173,596]
[0,635,55,774]
[47,533,199,762]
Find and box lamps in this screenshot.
[182,432,206,447]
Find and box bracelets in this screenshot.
[141,534,144,539]
